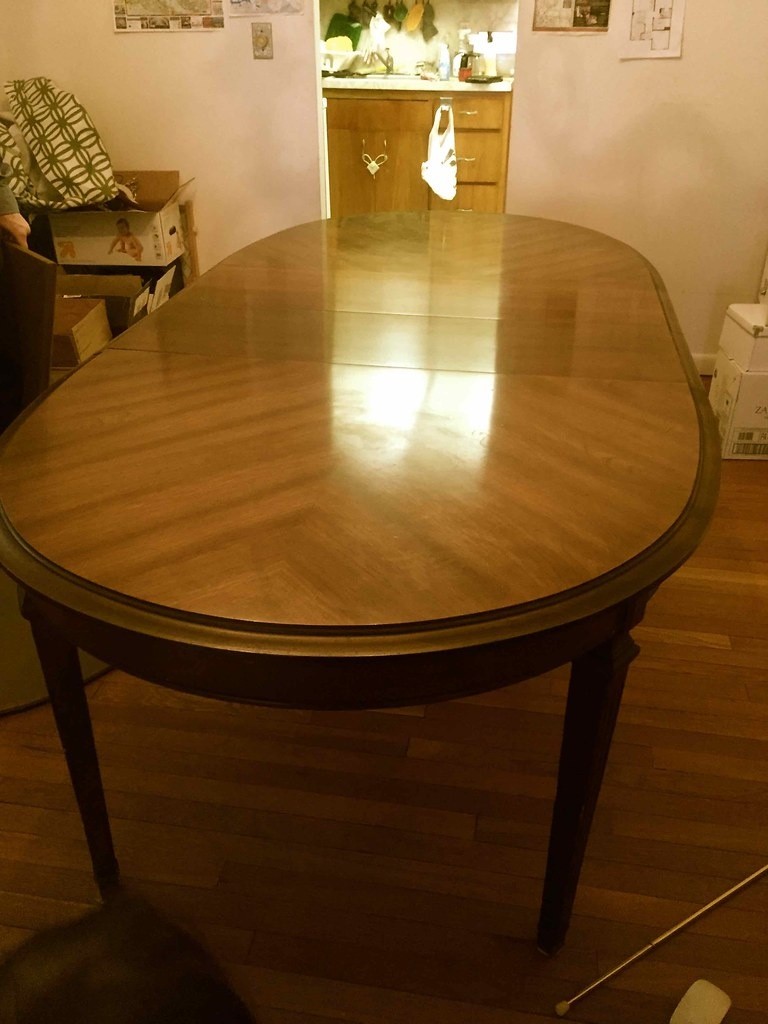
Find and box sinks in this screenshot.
[345,74,420,80]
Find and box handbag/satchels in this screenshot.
[422,106,457,201]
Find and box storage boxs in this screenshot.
[49,170,199,407]
[709,302,768,461]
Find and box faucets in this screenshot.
[374,47,393,73]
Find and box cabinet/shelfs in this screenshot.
[322,90,433,220]
[434,97,505,212]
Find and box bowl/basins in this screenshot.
[322,49,360,72]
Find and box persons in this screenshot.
[0,173,31,250]
[108,218,142,261]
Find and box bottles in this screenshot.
[467,50,474,67]
[485,39,497,76]
[453,22,471,77]
[440,44,450,80]
[472,54,482,76]
[457,67,471,81]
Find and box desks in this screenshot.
[0,215,723,953]
